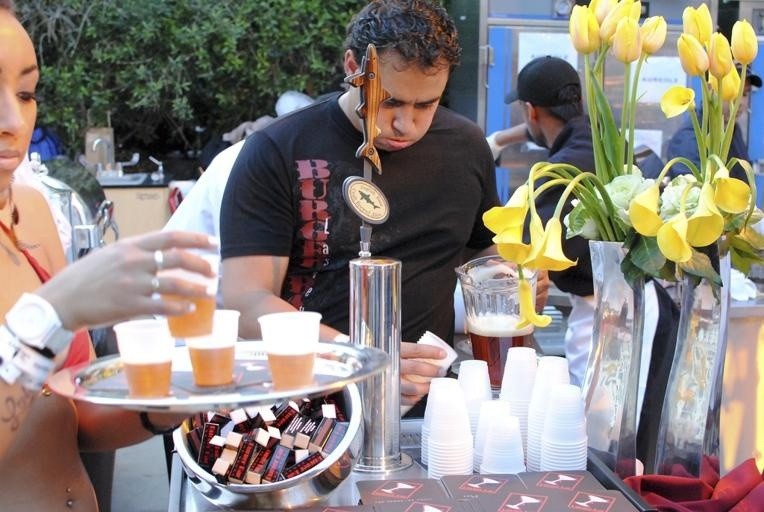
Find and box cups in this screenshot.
[111,237,322,402]
[454,258,536,386]
[405,330,589,482]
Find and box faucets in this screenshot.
[91,137,111,172]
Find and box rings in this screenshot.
[154,251,162,272]
[151,276,159,294]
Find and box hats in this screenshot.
[736,63,762,88]
[504,56,581,106]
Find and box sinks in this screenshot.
[95,172,148,185]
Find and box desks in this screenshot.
[95,171,175,245]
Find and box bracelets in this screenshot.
[141,413,182,434]
[0,326,55,391]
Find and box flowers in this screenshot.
[482,0,764,331]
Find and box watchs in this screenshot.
[5,292,75,355]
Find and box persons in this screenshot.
[159,1,762,418]
[0,0,220,512]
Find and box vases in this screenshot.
[582,239,732,490]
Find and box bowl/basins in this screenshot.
[171,383,366,508]
